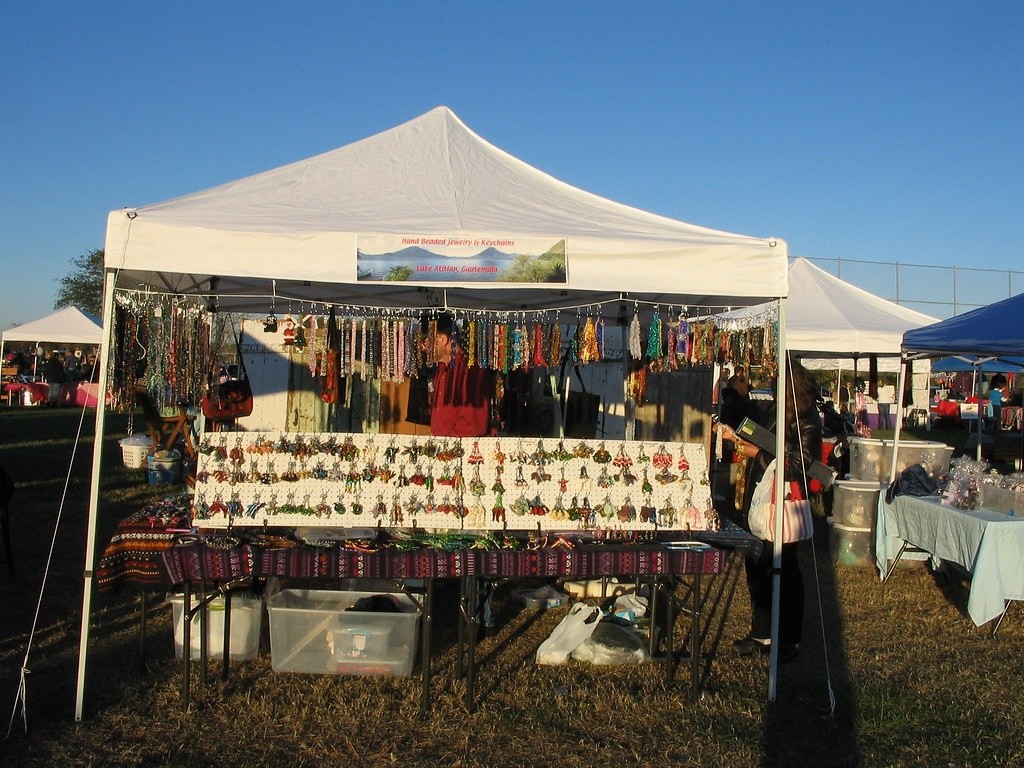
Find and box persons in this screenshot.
[875,379,893,431]
[989,383,1008,433]
[714,368,730,405]
[840,378,850,436]
[8,349,100,409]
[727,365,743,389]
[404,311,511,638]
[713,358,823,661]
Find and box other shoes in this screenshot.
[732,639,770,656]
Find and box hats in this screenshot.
[417,313,452,340]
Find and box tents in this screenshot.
[75,107,788,722]
[931,354,1024,434]
[889,292,1024,490]
[688,256,942,430]
[801,358,931,430]
[0,306,116,411]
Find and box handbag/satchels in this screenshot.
[201,379,254,421]
[541,348,600,439]
[748,459,814,543]
[536,602,604,667]
[316,349,341,404]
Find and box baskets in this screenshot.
[121,444,162,470]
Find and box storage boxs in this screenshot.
[119,440,152,470]
[827,436,957,569]
[978,481,1024,517]
[167,590,264,663]
[266,589,427,677]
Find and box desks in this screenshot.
[7,382,49,406]
[98,500,763,706]
[49,381,149,409]
[875,487,1024,636]
